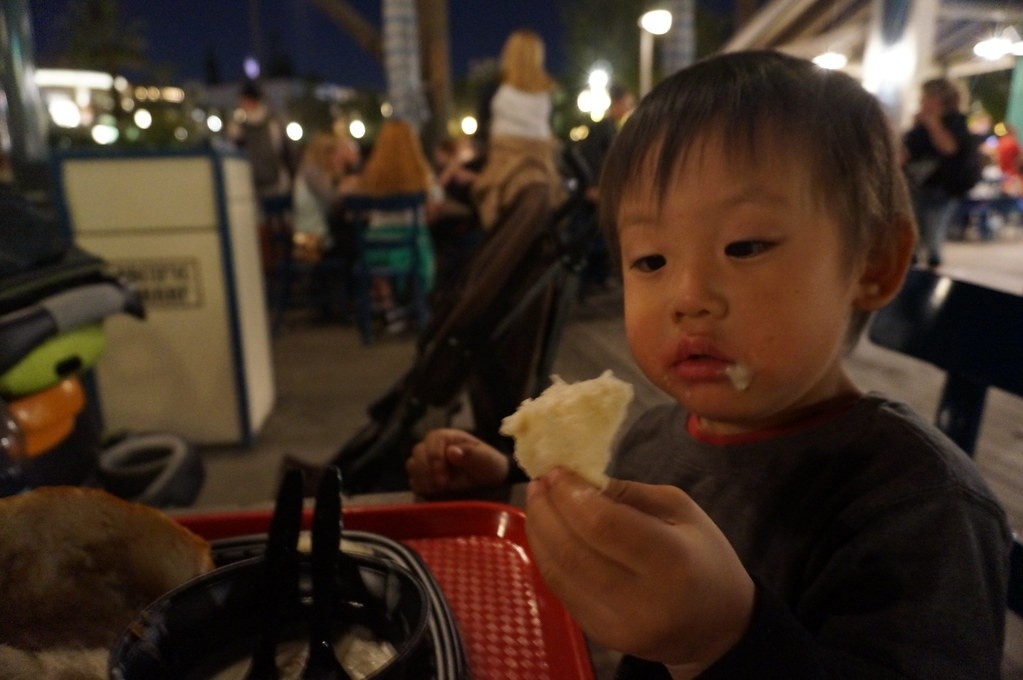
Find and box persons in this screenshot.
[226,31,631,338]
[406,50,1014,680]
[901,78,1023,273]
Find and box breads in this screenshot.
[0,489,217,680]
[501,372,634,493]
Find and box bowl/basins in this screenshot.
[107,551,430,680]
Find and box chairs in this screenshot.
[311,189,429,348]
[864,268,1023,628]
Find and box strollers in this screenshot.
[281,158,603,504]
